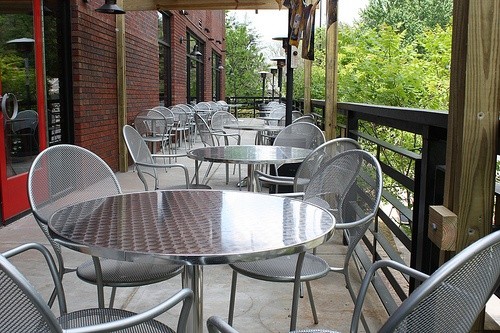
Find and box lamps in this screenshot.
[180,37,203,57]
[81,0,127,15]
[208,57,224,70]
[230,67,234,76]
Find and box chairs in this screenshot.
[0,99,500,333]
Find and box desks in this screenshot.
[257,117,285,126]
[262,108,271,111]
[186,143,319,193]
[47,186,338,333]
[224,124,287,188]
[261,105,272,108]
[257,111,300,114]
